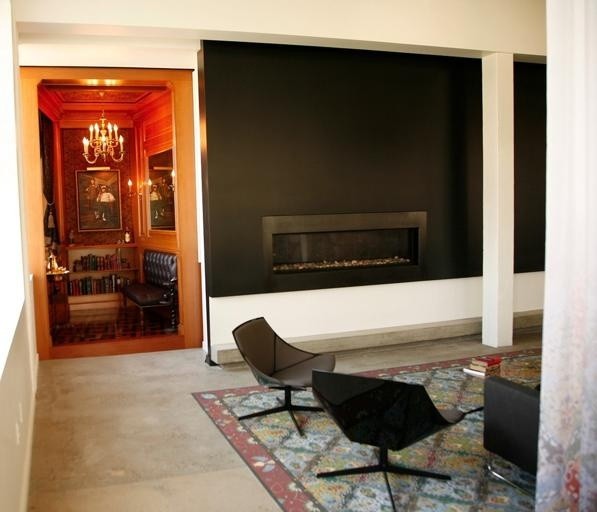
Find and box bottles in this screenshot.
[69,254,133,293]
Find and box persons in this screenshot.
[95,185,115,223]
[84,178,98,214]
[149,184,162,220]
[158,175,170,213]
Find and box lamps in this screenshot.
[83,110,124,164]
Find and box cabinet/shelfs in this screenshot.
[63,241,143,310]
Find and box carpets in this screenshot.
[192,350,540,512]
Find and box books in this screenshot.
[471,355,501,366]
[67,253,131,296]
[468,363,500,372]
[462,367,500,376]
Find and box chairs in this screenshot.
[230,315,336,437]
[311,370,466,512]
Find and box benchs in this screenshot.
[120,247,178,336]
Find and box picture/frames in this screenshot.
[140,140,177,238]
[75,167,126,235]
